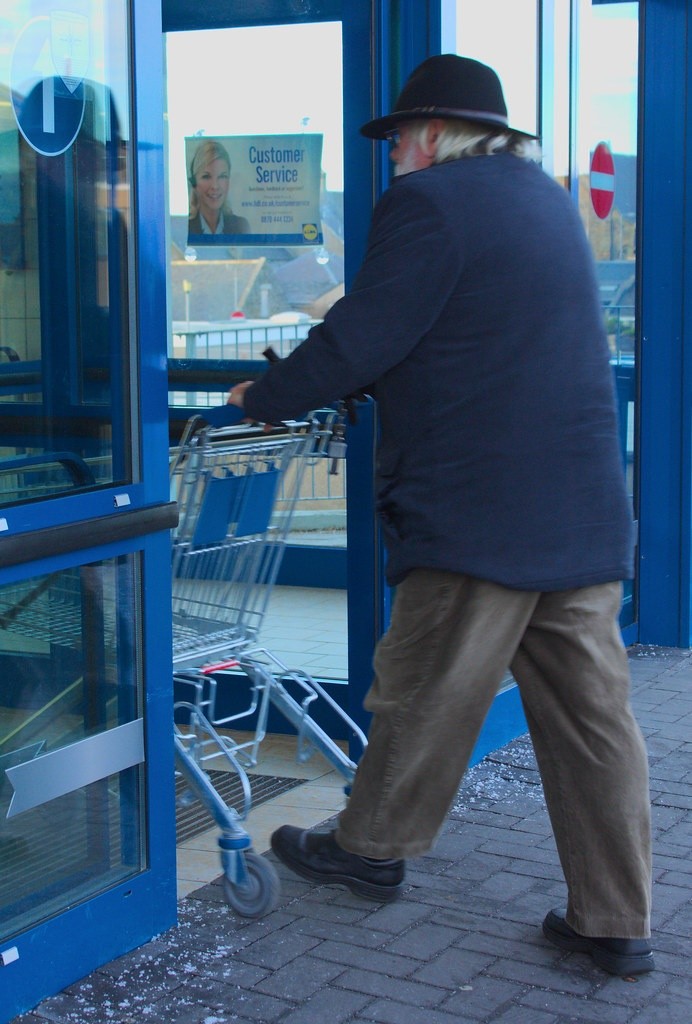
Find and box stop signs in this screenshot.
[587,143,614,220]
[232,311,246,321]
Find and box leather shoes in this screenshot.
[270,824,405,904]
[542,907,656,974]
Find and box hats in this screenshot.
[359,55,540,140]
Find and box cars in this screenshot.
[593,261,636,317]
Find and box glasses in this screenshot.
[382,118,427,147]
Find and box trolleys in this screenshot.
[0,406,368,920]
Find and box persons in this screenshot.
[188,141,251,237]
[228,53,657,980]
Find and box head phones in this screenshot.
[188,142,221,188]
